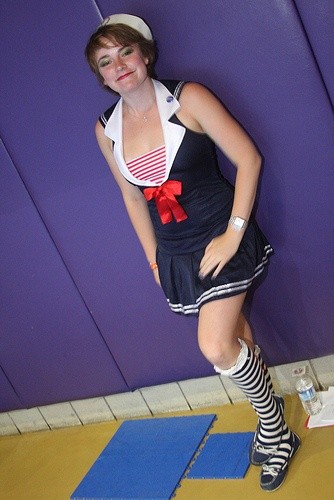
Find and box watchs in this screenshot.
[228,216,249,232]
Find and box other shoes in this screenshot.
[258,431,301,493]
[249,394,285,467]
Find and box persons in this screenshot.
[84,12,303,493]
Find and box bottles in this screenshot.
[292,366,322,416]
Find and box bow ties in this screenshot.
[143,180,190,223]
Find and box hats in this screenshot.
[98,14,155,42]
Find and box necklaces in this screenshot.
[129,98,158,122]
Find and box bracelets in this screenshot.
[151,263,157,271]
